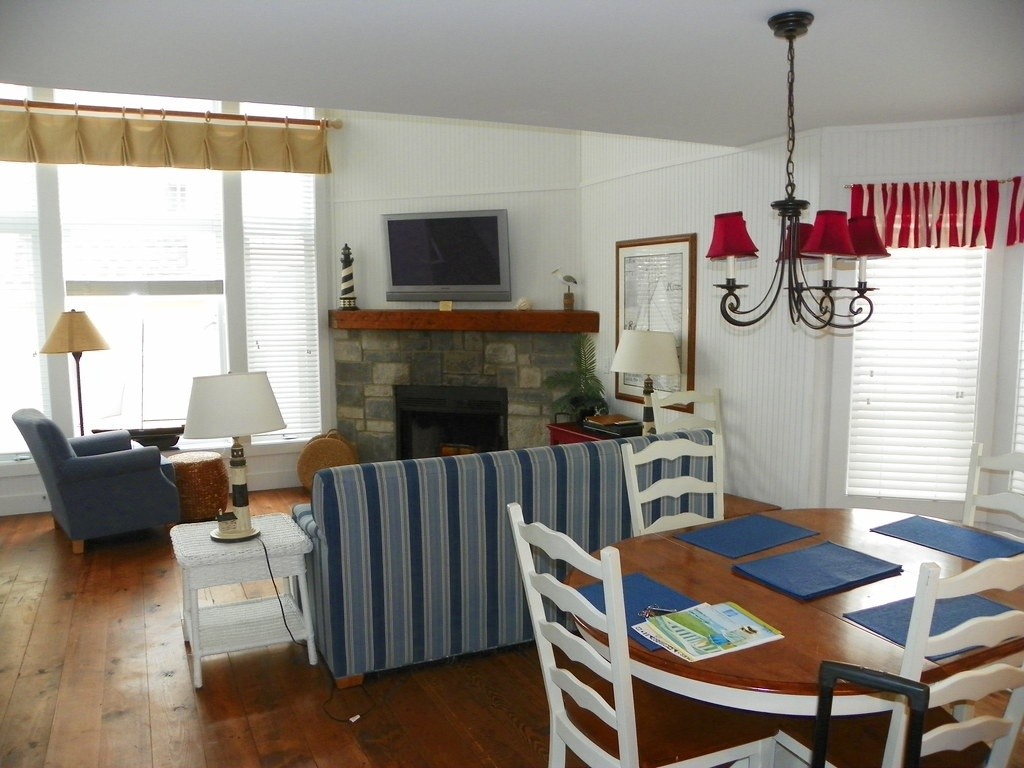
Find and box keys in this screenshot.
[637,604,677,619]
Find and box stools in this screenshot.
[170,452,229,522]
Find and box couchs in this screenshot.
[11,408,180,553]
[284,429,715,690]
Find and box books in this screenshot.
[585,414,634,426]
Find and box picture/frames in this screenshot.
[616,233,697,413]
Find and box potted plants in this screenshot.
[541,332,609,428]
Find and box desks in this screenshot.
[545,422,612,445]
[169,513,318,689]
[567,508,1024,724]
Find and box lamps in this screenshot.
[40,309,110,436]
[706,12,891,330]
[183,371,286,543]
[610,329,681,437]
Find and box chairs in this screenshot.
[506,502,781,768]
[773,553,1024,768]
[650,388,782,519]
[621,434,724,537]
[963,442,1024,545]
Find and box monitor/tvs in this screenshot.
[380,209,512,302]
